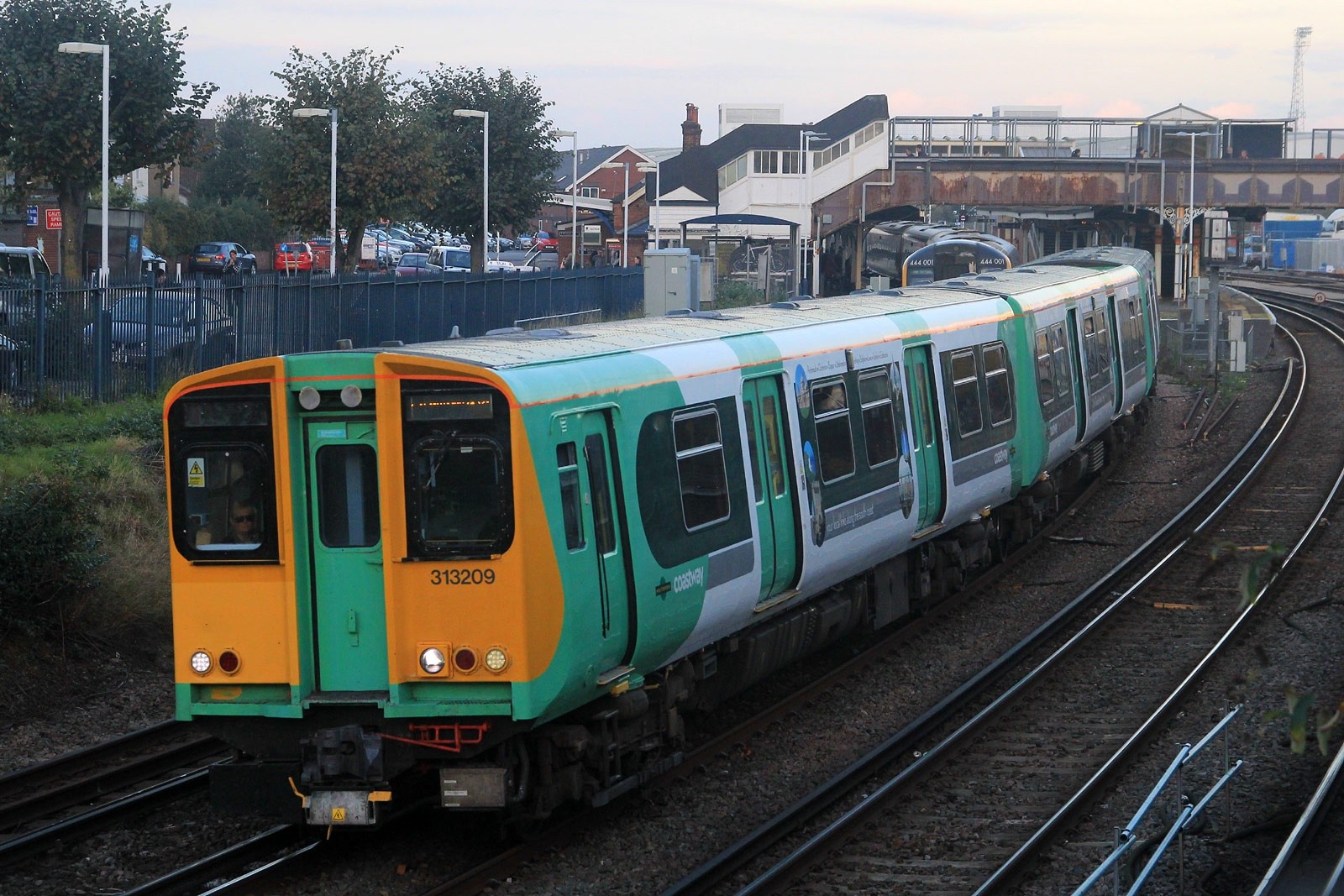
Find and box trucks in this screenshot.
[1319,209,1344,240]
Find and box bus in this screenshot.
[1242,235,1264,264]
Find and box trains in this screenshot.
[162,246,1161,836]
[865,218,1018,285]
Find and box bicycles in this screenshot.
[732,238,785,273]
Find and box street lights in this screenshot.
[454,109,490,271]
[548,130,577,269]
[290,108,337,277]
[599,163,628,268]
[797,129,832,297]
[1164,132,1226,277]
[58,42,109,314]
[636,161,659,250]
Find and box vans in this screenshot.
[188,241,257,277]
[0,245,63,336]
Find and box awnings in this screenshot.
[618,218,649,236]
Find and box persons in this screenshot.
[1238,150,1249,159]
[1135,147,1145,158]
[155,268,182,287]
[983,150,990,157]
[1151,151,1159,158]
[1222,147,1234,160]
[592,250,605,267]
[1315,152,1325,159]
[635,256,641,266]
[560,252,579,270]
[916,144,927,156]
[612,250,619,268]
[906,148,914,158]
[819,244,842,289]
[229,500,263,543]
[1071,149,1081,158]
[380,266,389,275]
[222,250,243,318]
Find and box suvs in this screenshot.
[273,242,319,272]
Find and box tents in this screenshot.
[678,213,799,298]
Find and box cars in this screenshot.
[532,230,558,252]
[337,220,514,262]
[82,288,235,375]
[515,232,533,250]
[0,334,23,392]
[395,251,430,276]
[424,245,541,272]
[142,246,166,284]
